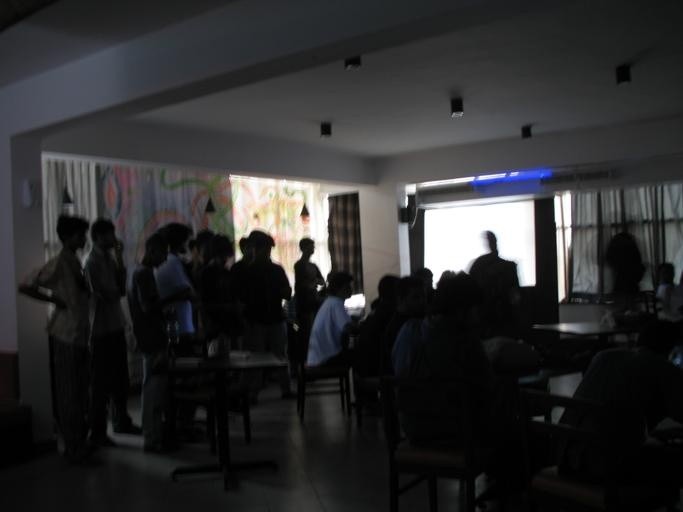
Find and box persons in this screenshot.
[125,223,682,511]
[17,215,105,468]
[83,218,143,450]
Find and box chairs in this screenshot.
[639,262,675,317]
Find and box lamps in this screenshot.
[522,126,532,139]
[344,56,361,72]
[320,122,331,138]
[449,97,464,119]
[616,63,631,86]
